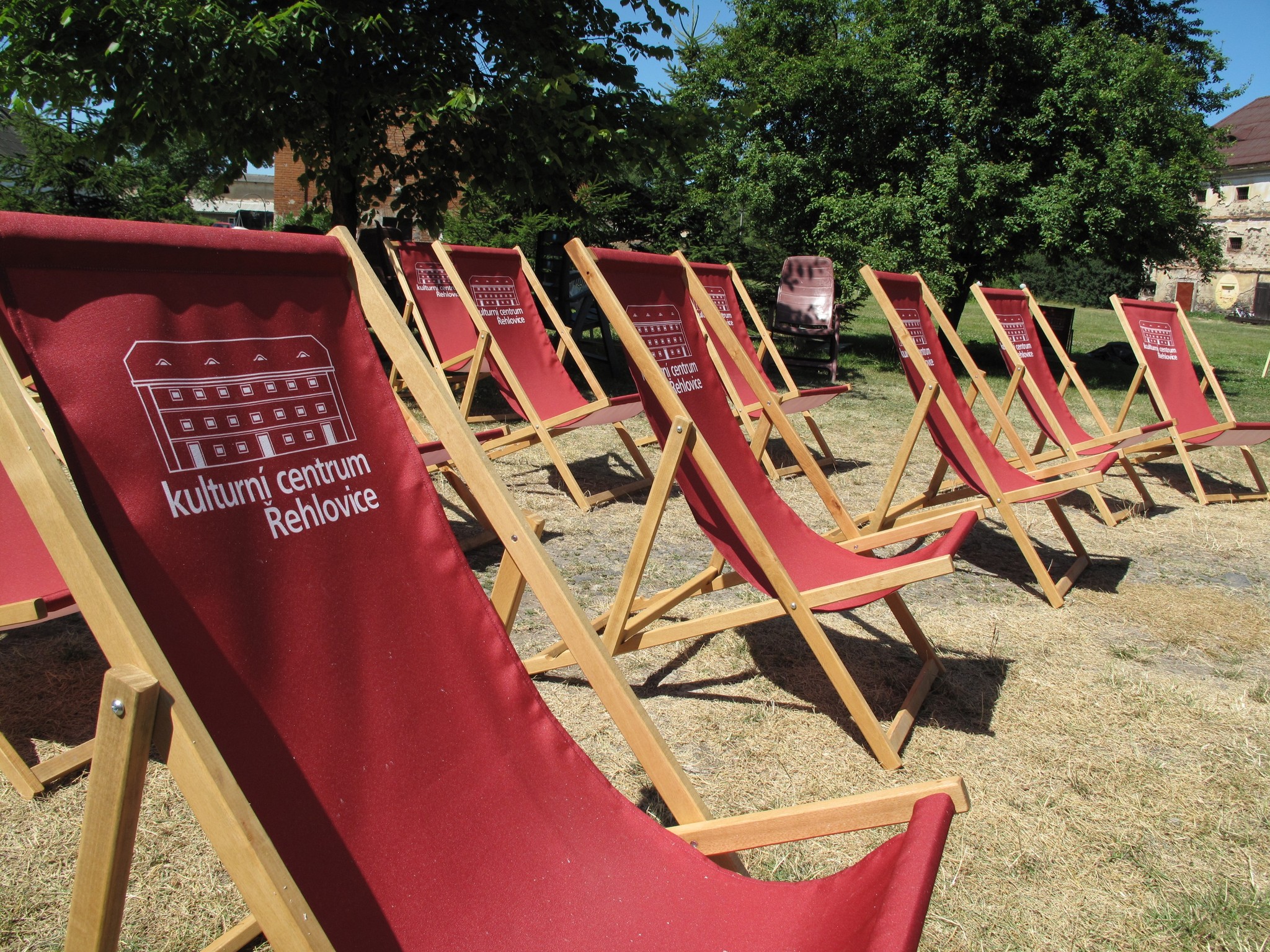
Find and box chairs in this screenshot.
[968,284,1173,528]
[1107,292,1269,508]
[816,265,1126,610]
[0,199,982,952]
